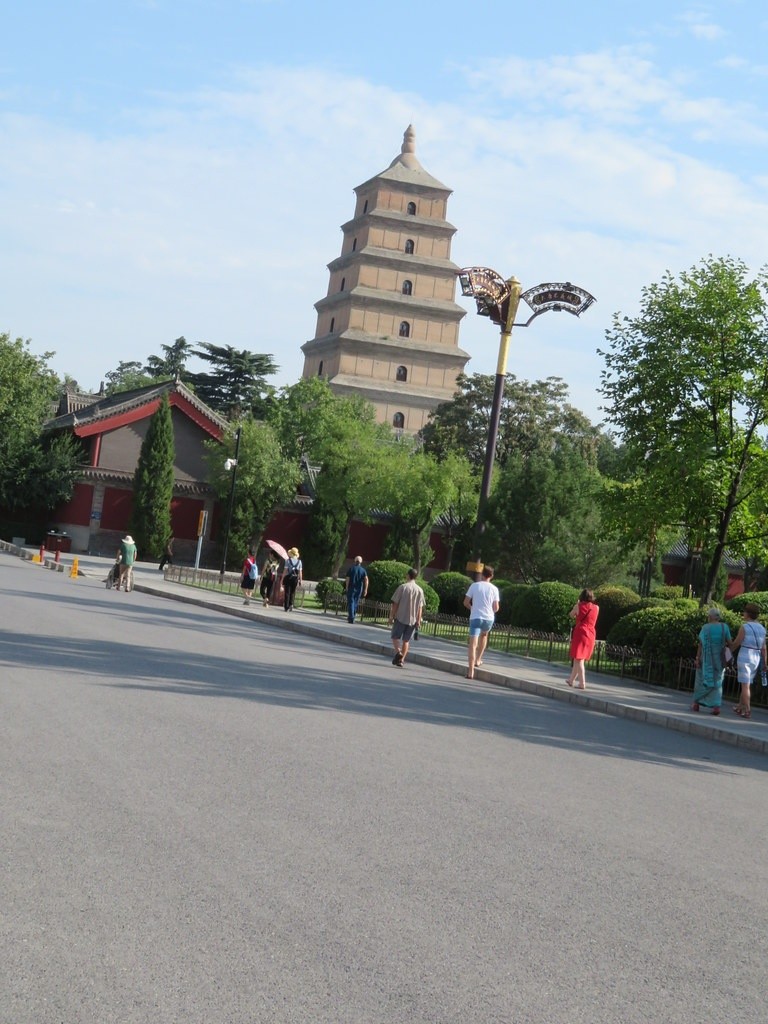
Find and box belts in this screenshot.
[741,646,762,650]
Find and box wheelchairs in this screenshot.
[105,560,135,593]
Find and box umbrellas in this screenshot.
[265,540,289,560]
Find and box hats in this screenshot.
[288,547,298,558]
[355,556,362,563]
[121,536,135,545]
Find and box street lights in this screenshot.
[457,265,598,584]
[217,423,243,583]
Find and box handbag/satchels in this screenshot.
[721,646,733,668]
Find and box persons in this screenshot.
[104,535,138,591]
[260,550,279,607]
[240,549,256,606]
[345,555,368,623]
[389,569,426,667]
[729,603,768,717]
[282,547,302,612]
[463,565,500,679]
[566,589,600,689]
[689,608,732,715]
[159,536,175,570]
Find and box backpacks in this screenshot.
[266,559,279,581]
[287,559,301,584]
[246,558,258,580]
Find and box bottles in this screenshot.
[761,669,767,686]
[414,627,419,640]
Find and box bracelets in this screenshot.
[696,657,699,660]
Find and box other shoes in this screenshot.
[286,605,294,612]
[566,679,573,686]
[243,596,252,605]
[475,660,483,667]
[125,587,130,592]
[577,684,585,689]
[732,706,741,714]
[347,618,355,624]
[264,598,269,608]
[466,673,473,679]
[742,711,752,717]
[690,704,699,711]
[116,586,121,591]
[398,660,404,667]
[392,652,404,665]
[712,709,721,716]
